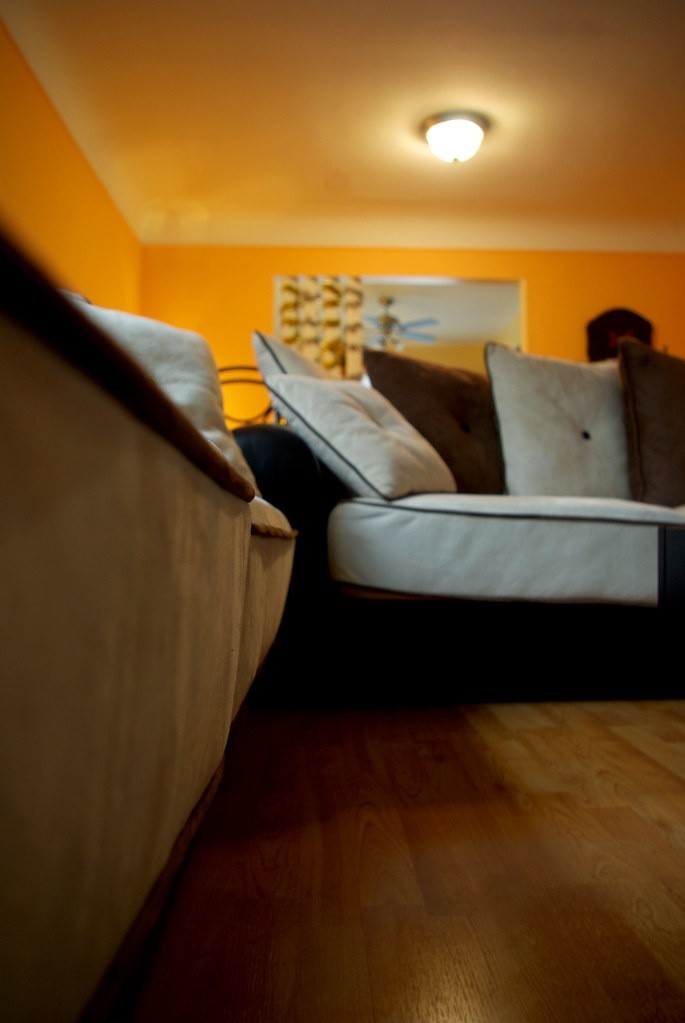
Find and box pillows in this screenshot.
[618,336,685,509]
[253,330,318,374]
[362,348,504,495]
[484,341,632,500]
[263,373,457,502]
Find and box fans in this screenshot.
[364,296,436,342]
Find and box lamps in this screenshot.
[422,112,488,164]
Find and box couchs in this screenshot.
[230,423,684,707]
[1,213,298,1023]
